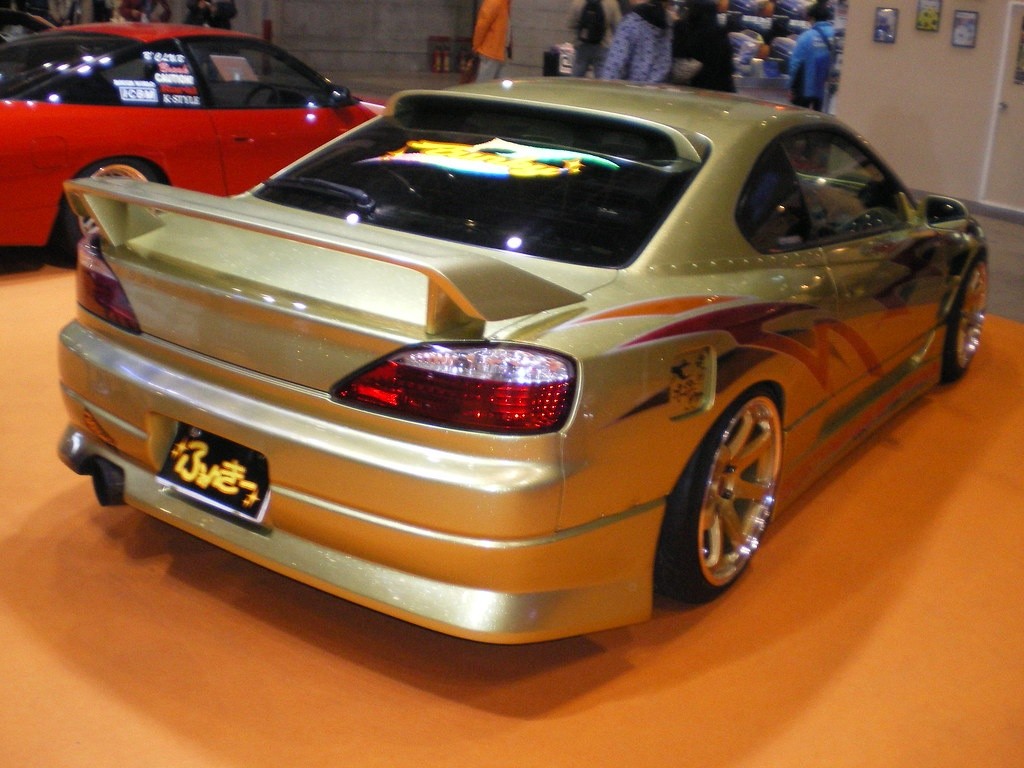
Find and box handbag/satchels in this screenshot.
[671,55,703,85]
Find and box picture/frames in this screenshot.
[951,9,979,48]
[873,7,900,45]
[915,0,943,32]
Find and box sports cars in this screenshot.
[0,24,378,269]
[56,78,990,643]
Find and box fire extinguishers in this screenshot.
[458,47,467,72]
[434,45,450,74]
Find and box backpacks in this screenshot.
[577,0,605,44]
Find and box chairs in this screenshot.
[701,143,813,243]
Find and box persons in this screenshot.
[471,0,512,82]
[0,0,172,24]
[784,0,836,111]
[573,0,736,92]
[184,0,237,30]
